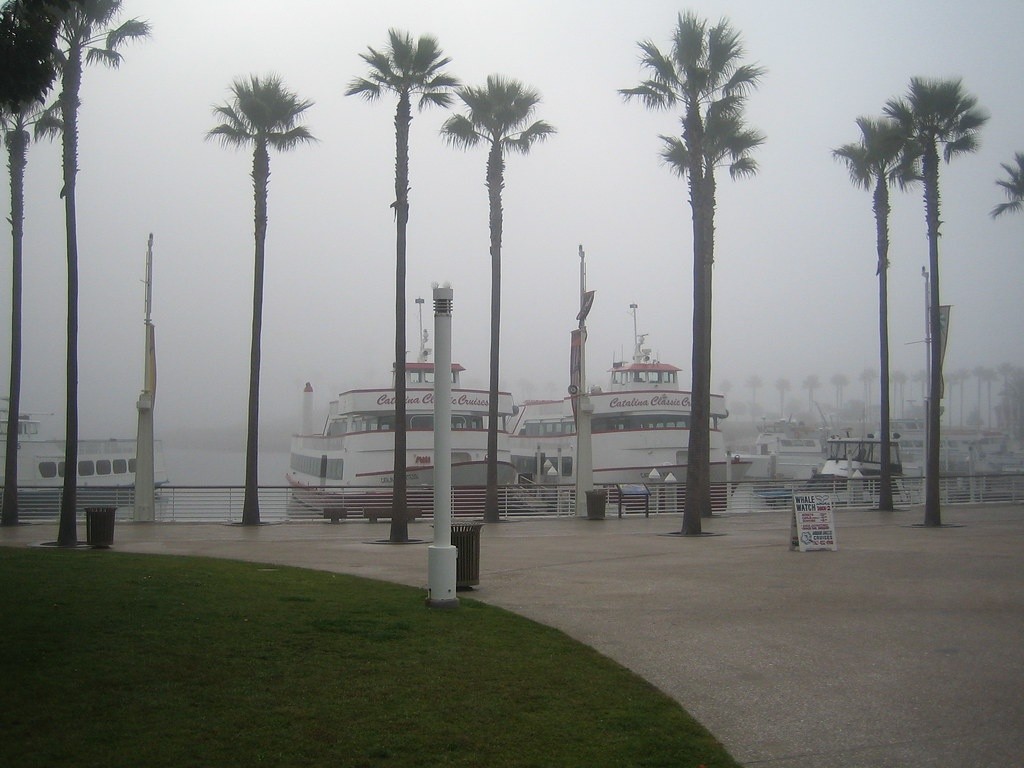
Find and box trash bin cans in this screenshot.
[84,505,118,546]
[584,489,607,520]
[430,522,486,587]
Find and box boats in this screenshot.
[286,295,521,517]
[0,410,172,514]
[727,410,1024,506]
[501,304,754,514]
